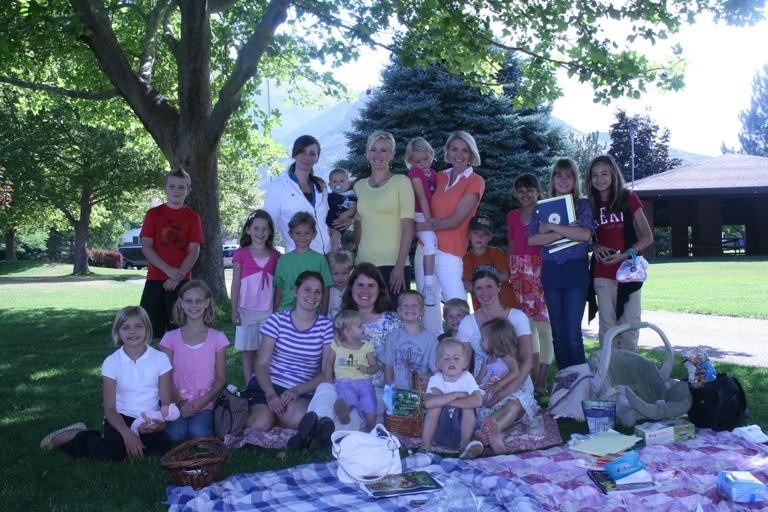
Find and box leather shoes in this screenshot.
[40,422,86,449]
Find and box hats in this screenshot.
[468,215,494,235]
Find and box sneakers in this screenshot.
[287,411,334,452]
[459,440,483,458]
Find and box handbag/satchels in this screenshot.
[617,257,648,284]
[331,423,402,485]
[214,383,248,445]
[689,372,747,428]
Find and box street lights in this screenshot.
[624,126,640,196]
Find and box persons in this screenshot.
[39,305,173,463]
[140,167,205,339]
[586,156,653,352]
[158,279,230,444]
[525,159,596,371]
[230,130,553,460]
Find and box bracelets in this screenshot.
[177,399,184,406]
[557,233,561,239]
[633,242,641,253]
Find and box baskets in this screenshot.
[161,437,227,490]
[384,371,426,438]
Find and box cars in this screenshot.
[120,241,147,270]
[221,249,239,269]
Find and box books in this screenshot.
[604,449,652,484]
[537,192,584,254]
[587,470,656,495]
